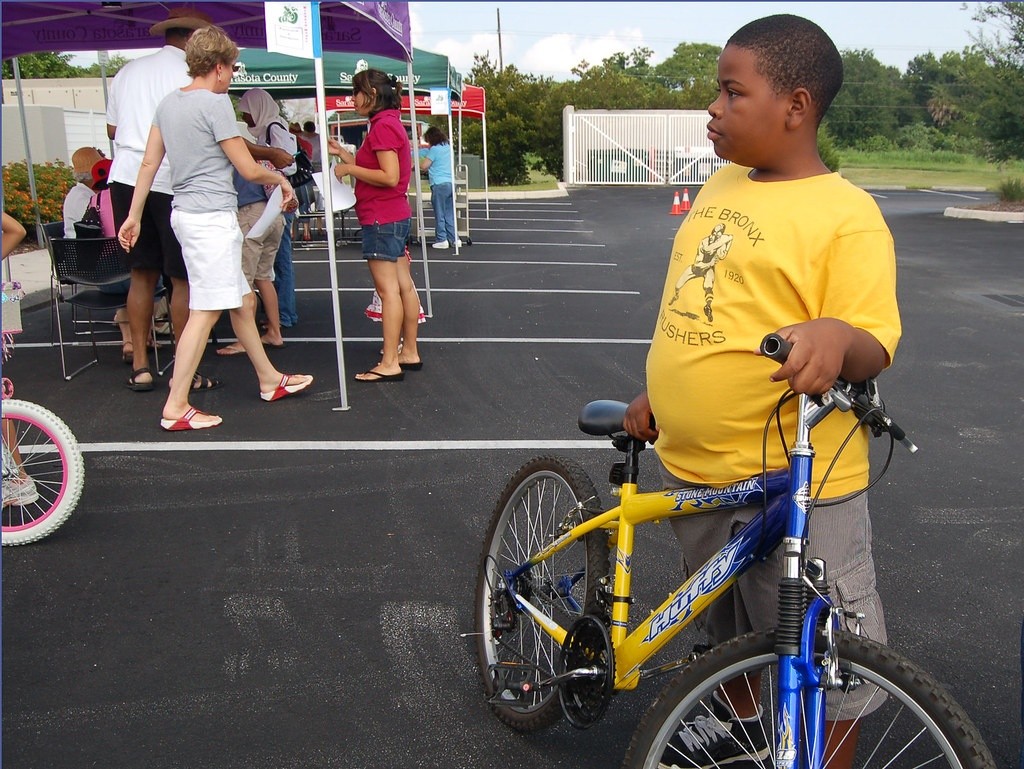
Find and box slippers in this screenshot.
[159,404,223,431]
[353,368,406,384]
[402,357,424,372]
[260,373,314,401]
[216,345,247,356]
[259,338,288,348]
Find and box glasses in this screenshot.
[223,61,239,73]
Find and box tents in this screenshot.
[316,83,490,220]
[1,0,433,410]
[223,46,463,256]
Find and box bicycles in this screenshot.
[473,332,999,769]
[1,377,89,551]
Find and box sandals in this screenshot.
[146,339,162,350]
[168,368,224,390]
[126,371,153,390]
[121,343,133,362]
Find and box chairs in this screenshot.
[290,180,363,250]
[38,220,173,382]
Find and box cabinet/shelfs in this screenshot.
[408,165,472,246]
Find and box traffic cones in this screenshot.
[670,191,684,215]
[680,188,691,211]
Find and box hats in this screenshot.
[72,147,105,173]
[149,6,213,37]
[91,159,115,186]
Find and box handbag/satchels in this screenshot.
[266,122,315,188]
[74,197,104,269]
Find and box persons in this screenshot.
[416,127,463,249]
[622,14,902,769]
[0,210,40,509]
[328,69,423,383]
[106,8,224,393]
[118,27,313,431]
[238,88,298,328]
[67,147,284,360]
[286,121,323,240]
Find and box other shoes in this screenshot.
[260,318,286,331]
[304,235,310,241]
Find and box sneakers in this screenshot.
[452,240,463,248]
[657,692,770,769]
[1,476,38,509]
[432,241,449,249]
